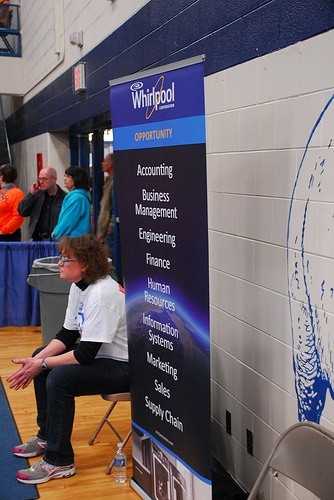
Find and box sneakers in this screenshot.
[14,436,48,457]
[17,459,75,484]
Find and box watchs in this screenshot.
[41,357,49,372]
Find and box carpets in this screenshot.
[0,376,41,500]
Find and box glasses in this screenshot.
[59,254,84,264]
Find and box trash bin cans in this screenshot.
[28,257,112,347]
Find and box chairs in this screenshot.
[88,394,133,474]
[247,422,334,500]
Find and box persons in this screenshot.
[97,153,114,268]
[50,166,93,242]
[0,164,25,242]
[6,236,132,484]
[17,167,68,241]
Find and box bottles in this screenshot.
[113,443,127,484]
